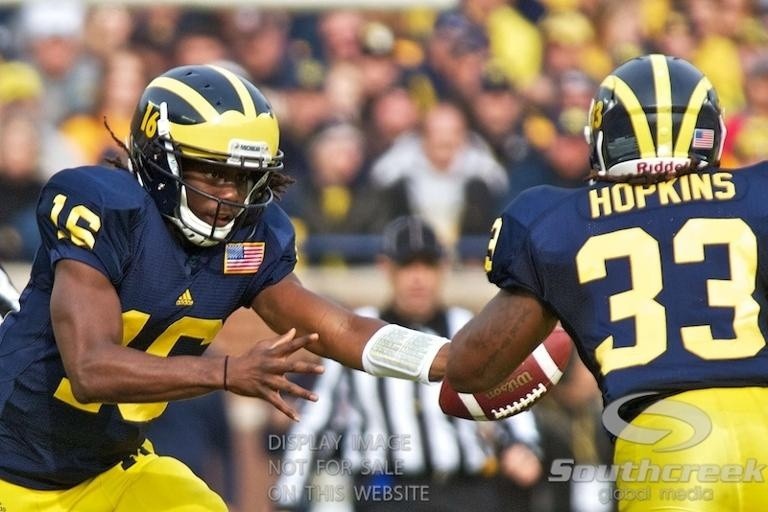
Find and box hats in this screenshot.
[381,218,445,261]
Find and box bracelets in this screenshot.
[520,441,545,470]
[223,354,231,391]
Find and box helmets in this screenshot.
[128,64,284,248]
[582,54,728,186]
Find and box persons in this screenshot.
[1,2,768,263]
[446,55,768,512]
[0,62,450,512]
[272,217,544,510]
